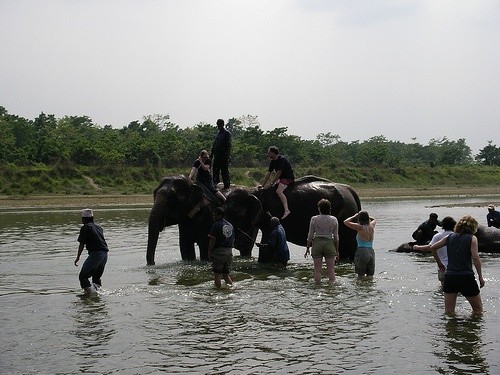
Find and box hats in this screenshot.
[82,209,93,217]
[488,204,494,209]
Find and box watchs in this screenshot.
[270,181,274,185]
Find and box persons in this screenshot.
[74,209,109,297]
[430,215,485,316]
[343,211,377,276]
[207,207,236,287]
[413,217,456,286]
[487,204,500,229]
[209,119,231,190]
[259,145,294,219]
[255,211,290,267]
[189,150,227,201]
[304,199,339,282]
[412,213,442,244]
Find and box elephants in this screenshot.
[146,174,362,265]
